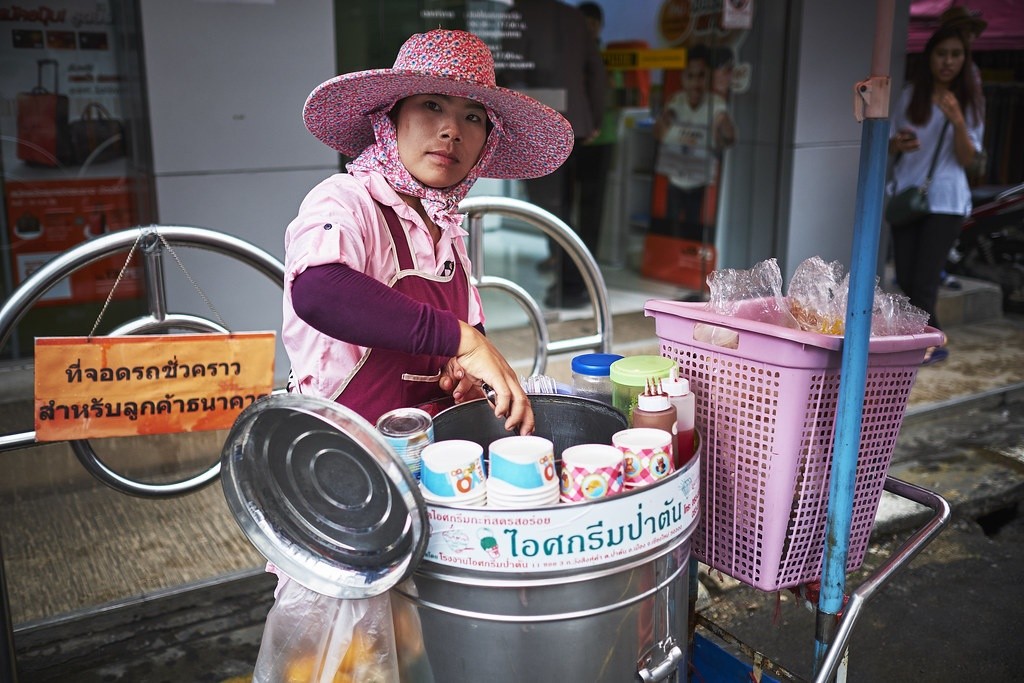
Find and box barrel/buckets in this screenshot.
[219,393,702,680]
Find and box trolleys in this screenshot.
[686,471,950,683]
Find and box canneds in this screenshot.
[376,407,434,485]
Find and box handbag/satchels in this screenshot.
[249,555,437,683]
[883,183,931,230]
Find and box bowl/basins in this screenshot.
[418,428,677,511]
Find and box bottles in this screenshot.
[632,376,677,468]
[660,367,696,467]
[377,406,435,482]
[610,354,679,430]
[570,353,628,406]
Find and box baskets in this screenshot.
[643,293,949,596]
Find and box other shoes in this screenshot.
[918,345,948,365]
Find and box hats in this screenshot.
[301,28,575,178]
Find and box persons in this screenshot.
[886,26,985,364]
[502,0,608,310]
[661,47,734,235]
[282,28,575,436]
[930,3,989,290]
[578,0,625,259]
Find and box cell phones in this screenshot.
[900,128,919,150]
